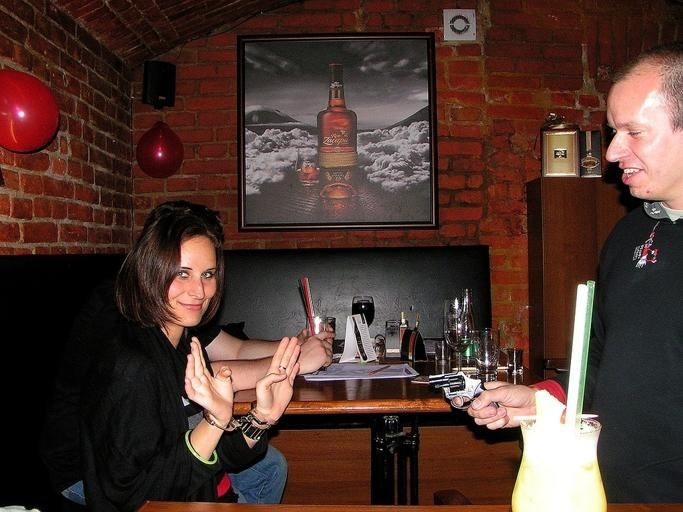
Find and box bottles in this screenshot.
[316,64,359,198]
[458,288,477,366]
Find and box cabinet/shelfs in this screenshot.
[525,173,627,373]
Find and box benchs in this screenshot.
[211,242,525,504]
[1,234,158,511]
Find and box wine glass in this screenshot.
[443,300,476,378]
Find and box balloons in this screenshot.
[136,121,183,177]
[0,70,59,153]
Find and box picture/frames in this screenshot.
[232,28,441,233]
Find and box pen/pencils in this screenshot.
[367,365,391,376]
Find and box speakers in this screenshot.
[142,60,176,107]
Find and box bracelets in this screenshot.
[241,422,265,440]
[184,430,218,465]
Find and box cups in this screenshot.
[295,149,320,187]
[351,296,374,333]
[511,419,608,512]
[506,348,525,375]
[304,317,334,373]
[474,328,499,376]
[384,320,400,357]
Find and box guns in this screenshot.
[428,371,499,411]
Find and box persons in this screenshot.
[78,213,299,512]
[140,200,336,394]
[451,46,683,503]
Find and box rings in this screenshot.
[279,365,286,370]
